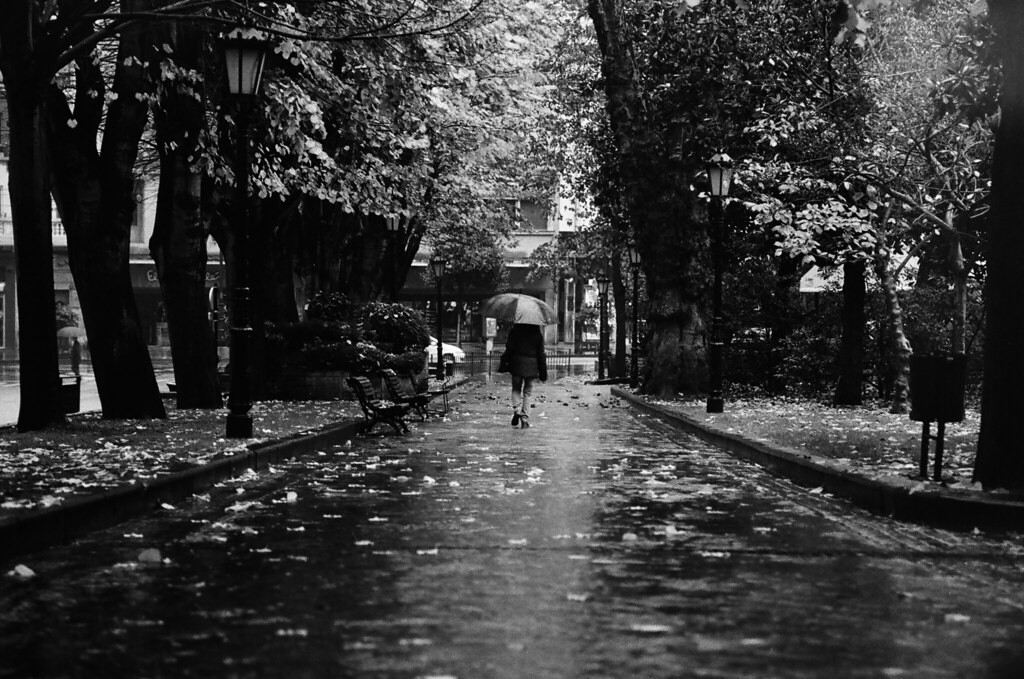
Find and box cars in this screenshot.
[425,336,466,365]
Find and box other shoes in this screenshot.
[522,422,529,429]
[512,414,519,426]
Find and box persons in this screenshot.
[70,337,82,382]
[496,323,549,429]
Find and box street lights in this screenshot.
[214,0,284,440]
[704,139,736,413]
[430,248,448,382]
[597,270,612,379]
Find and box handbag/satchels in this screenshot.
[498,354,509,373]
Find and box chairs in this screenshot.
[382,369,432,423]
[406,369,451,417]
[347,376,409,435]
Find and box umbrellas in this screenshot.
[56,326,85,338]
[477,293,561,327]
